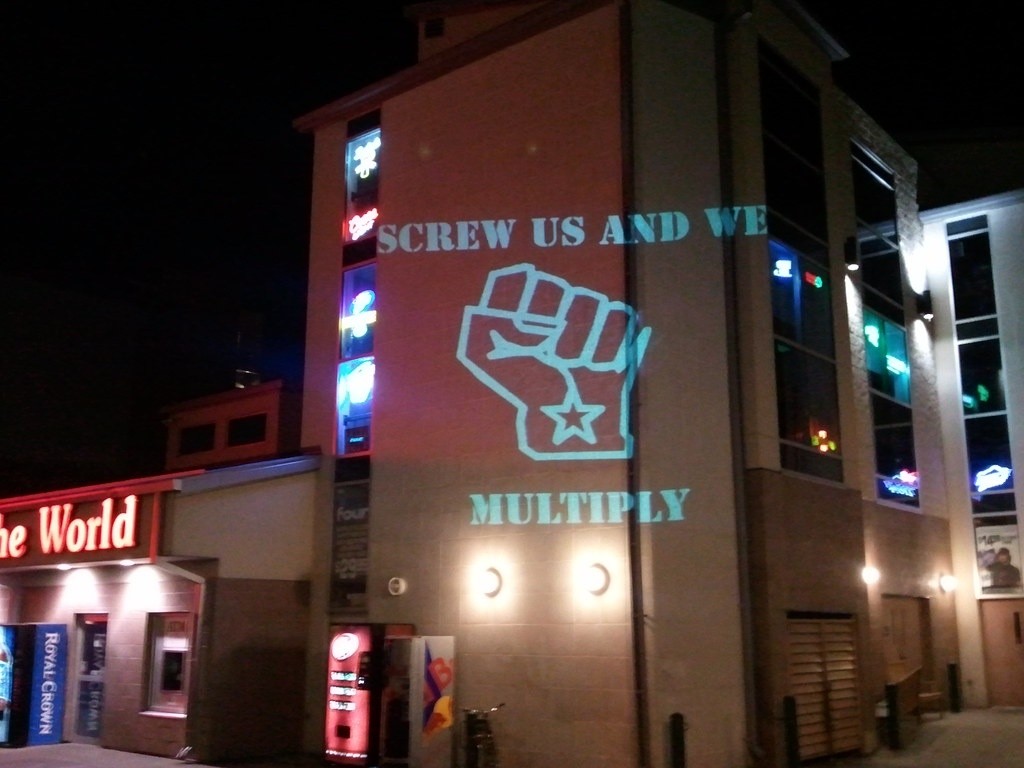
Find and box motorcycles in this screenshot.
[462,703,506,768]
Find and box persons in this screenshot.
[986,548,1020,586]
